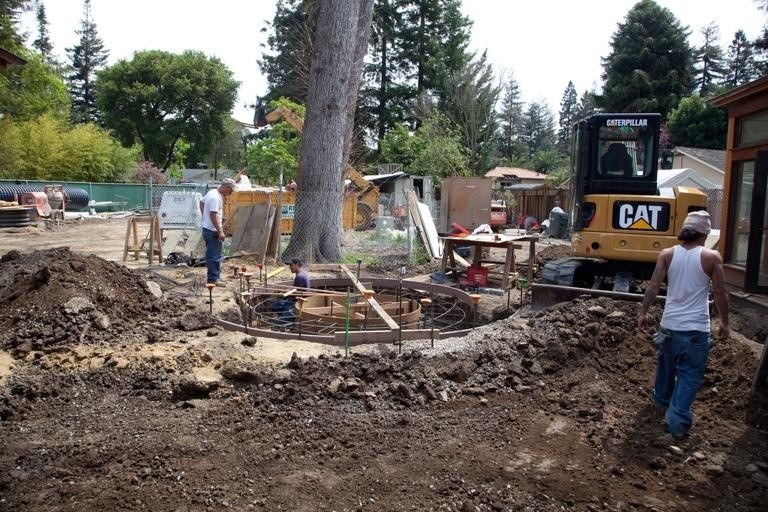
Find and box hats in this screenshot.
[222,178,239,193]
[285,256,304,265]
[682,210,712,234]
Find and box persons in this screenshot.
[637,208,731,442]
[271,257,311,321]
[200,177,236,288]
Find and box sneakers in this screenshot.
[651,388,690,441]
[206,276,228,287]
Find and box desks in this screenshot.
[438,233,538,294]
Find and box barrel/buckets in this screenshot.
[466,264,488,287]
[517,279,527,289]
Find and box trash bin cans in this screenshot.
[548,212,568,239]
[377,217,394,230]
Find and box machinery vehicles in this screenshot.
[531,108,708,310]
[223,105,380,235]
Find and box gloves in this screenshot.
[652,330,670,345]
